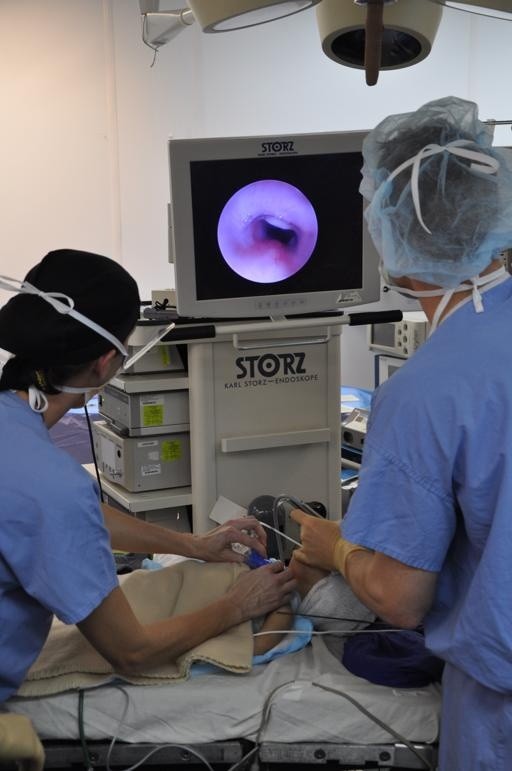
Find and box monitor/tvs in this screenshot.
[167,129,381,320]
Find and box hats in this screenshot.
[1,250,142,368]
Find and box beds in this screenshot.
[8,638,448,767]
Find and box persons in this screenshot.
[244,532,335,657]
[0,245,302,719]
[283,114,512,769]
